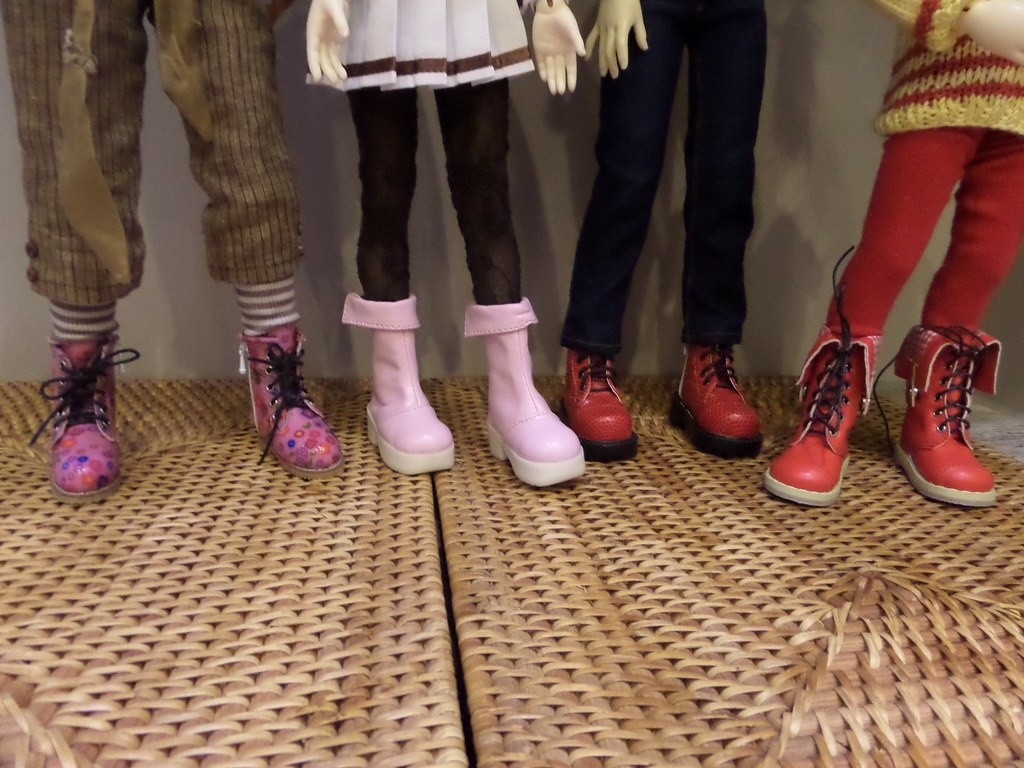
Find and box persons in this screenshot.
[763,0,1024,506]
[304,0,586,488]
[0,0,347,505]
[560,0,768,462]
